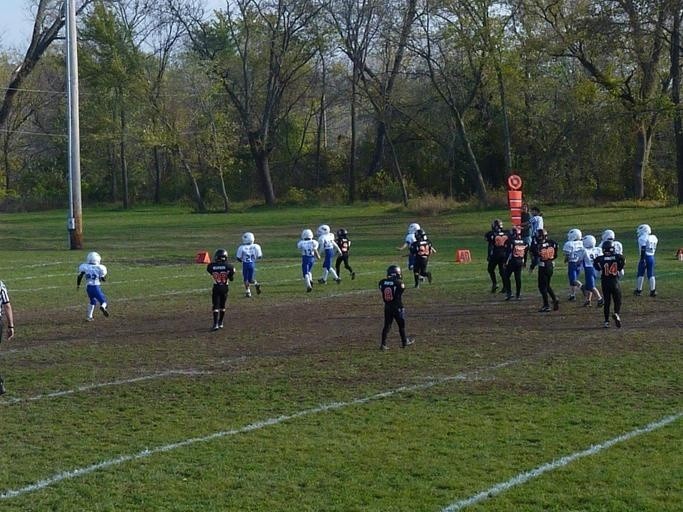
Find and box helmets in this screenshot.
[87,251,101,265]
[302,226,348,241]
[214,249,228,261]
[386,265,402,277]
[409,222,427,240]
[602,230,616,257]
[568,228,597,249]
[491,219,548,243]
[637,224,651,237]
[243,232,255,244]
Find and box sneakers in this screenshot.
[538,305,551,312]
[244,293,251,297]
[603,321,611,328]
[612,313,622,327]
[634,288,643,295]
[553,296,559,311]
[86,316,95,322]
[100,306,109,317]
[568,294,604,309]
[214,322,224,330]
[307,272,358,292]
[256,283,261,295]
[381,345,391,350]
[400,338,415,346]
[414,273,432,289]
[650,289,657,296]
[491,287,521,301]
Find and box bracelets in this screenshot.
[7,325,14,329]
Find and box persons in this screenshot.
[205,248,235,330]
[296,228,320,292]
[482,204,658,328]
[396,223,421,271]
[410,229,432,288]
[75,251,107,321]
[235,232,262,298]
[334,227,355,282]
[315,224,342,284]
[0,280,15,393]
[377,265,415,351]
